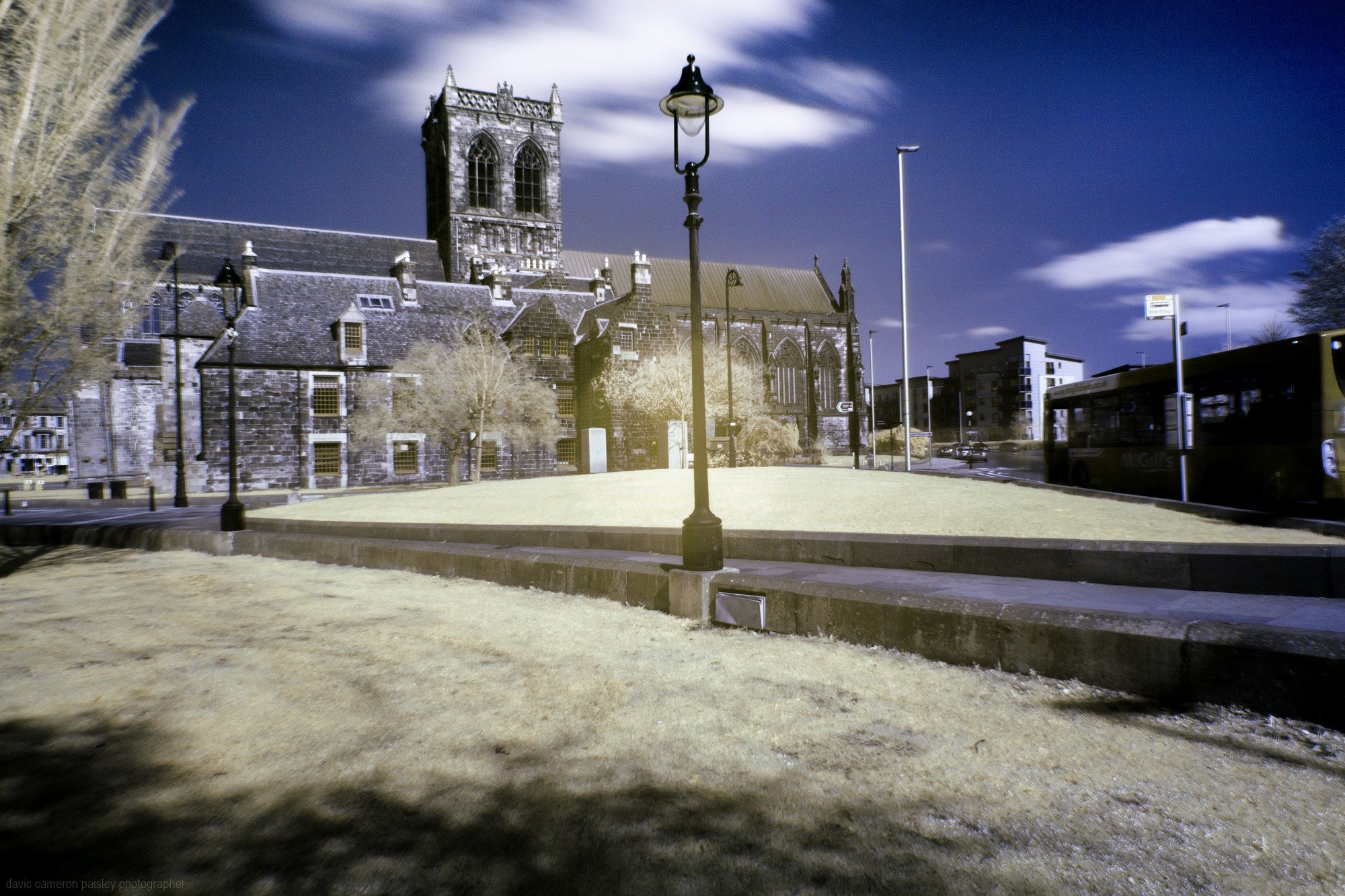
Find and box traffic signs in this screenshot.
[837,402,853,412]
[910,432,932,436]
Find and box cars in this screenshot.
[1000,442,1020,451]
[936,442,990,463]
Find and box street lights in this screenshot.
[153,239,189,507]
[926,365,935,466]
[966,411,973,469]
[869,330,879,467]
[659,53,725,569]
[1216,303,1232,350]
[725,269,743,467]
[899,385,904,426]
[1136,351,1146,368]
[212,257,247,531]
[896,145,922,471]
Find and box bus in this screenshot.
[1042,329,1345,511]
